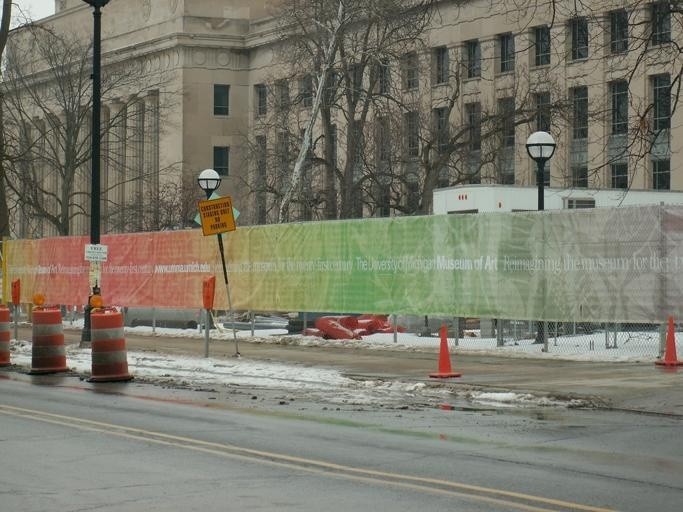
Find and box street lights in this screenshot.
[197,168,222,329]
[525,130,556,344]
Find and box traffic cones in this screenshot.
[655,315,683,366]
[429,325,462,377]
